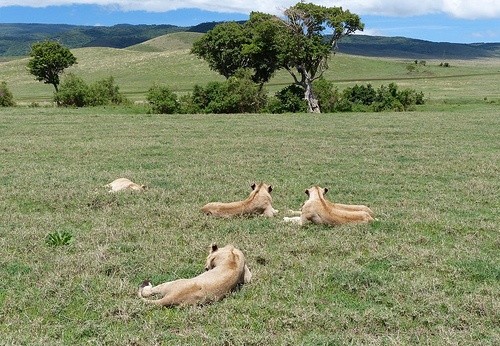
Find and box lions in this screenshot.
[200,183,278,218]
[283,186,374,227]
[104,177,145,195]
[137,242,251,310]
